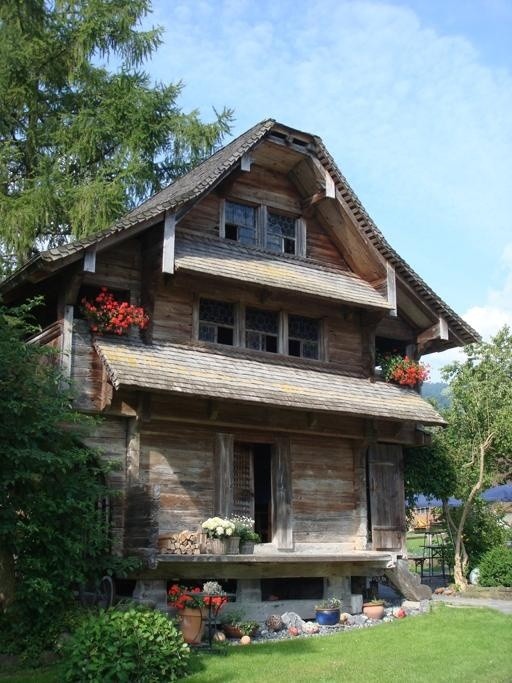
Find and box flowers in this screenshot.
[203,511,256,536]
[378,349,433,388]
[77,286,152,337]
[167,582,227,610]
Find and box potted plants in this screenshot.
[240,532,260,555]
[361,596,386,620]
[220,610,259,637]
[315,596,341,625]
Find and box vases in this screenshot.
[210,537,241,554]
[177,607,207,645]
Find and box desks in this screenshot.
[183,591,236,650]
